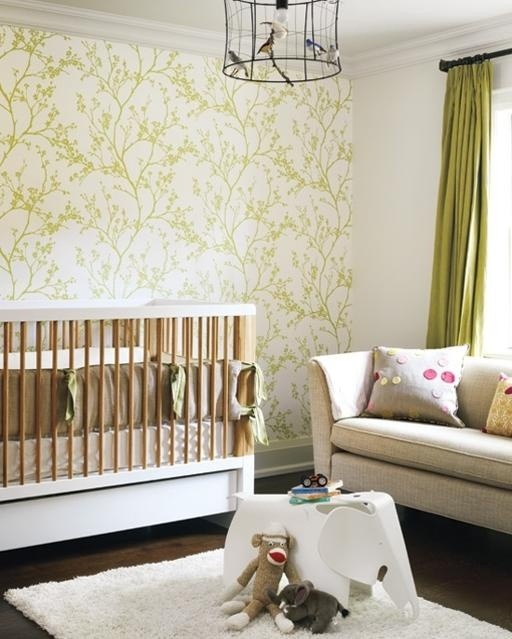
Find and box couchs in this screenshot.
[308,352,512,534]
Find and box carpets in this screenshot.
[3,547,511,639]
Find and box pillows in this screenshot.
[358,344,469,427]
[482,373,512,437]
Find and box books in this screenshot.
[291,478,344,492]
[288,497,331,505]
[285,489,342,501]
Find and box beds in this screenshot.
[0,301,269,553]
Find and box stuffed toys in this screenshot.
[214,522,303,635]
[265,580,351,635]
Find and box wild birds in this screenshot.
[306,39,328,56]
[256,21,288,56]
[326,45,339,67]
[227,49,249,77]
